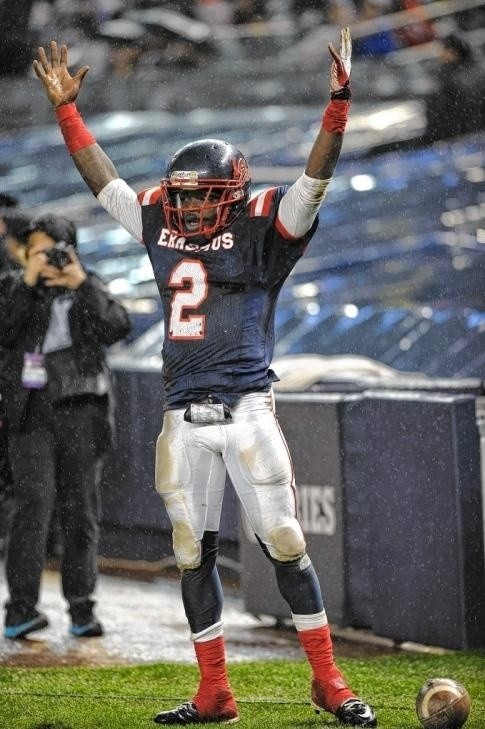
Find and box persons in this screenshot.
[0,193,58,559]
[32,25,378,723]
[420,34,484,144]
[2,0,433,70]
[0,214,132,638]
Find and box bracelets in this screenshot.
[323,95,349,134]
[55,103,93,155]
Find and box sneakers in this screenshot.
[154,698,240,724]
[6,600,49,638]
[309,675,378,727]
[70,598,104,637]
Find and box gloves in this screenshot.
[327,26,352,100]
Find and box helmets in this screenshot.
[160,138,249,236]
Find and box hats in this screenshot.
[22,212,78,248]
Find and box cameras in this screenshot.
[45,248,71,269]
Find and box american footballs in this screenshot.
[417,676,471,727]
